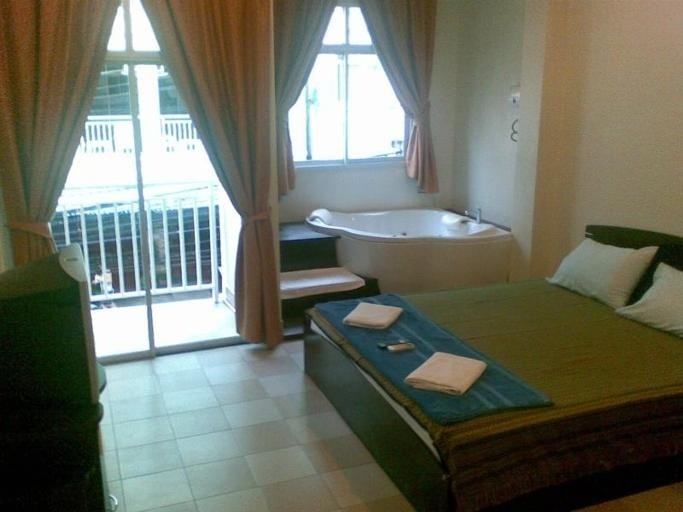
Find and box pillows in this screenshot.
[543,239,683,338]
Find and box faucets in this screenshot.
[464,208,482,224]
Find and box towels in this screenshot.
[402,349,489,397]
[278,266,404,330]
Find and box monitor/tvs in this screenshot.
[0,241,102,410]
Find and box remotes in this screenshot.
[376,339,408,348]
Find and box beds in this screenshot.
[303,224,683,511]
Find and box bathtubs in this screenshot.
[306,206,514,246]
